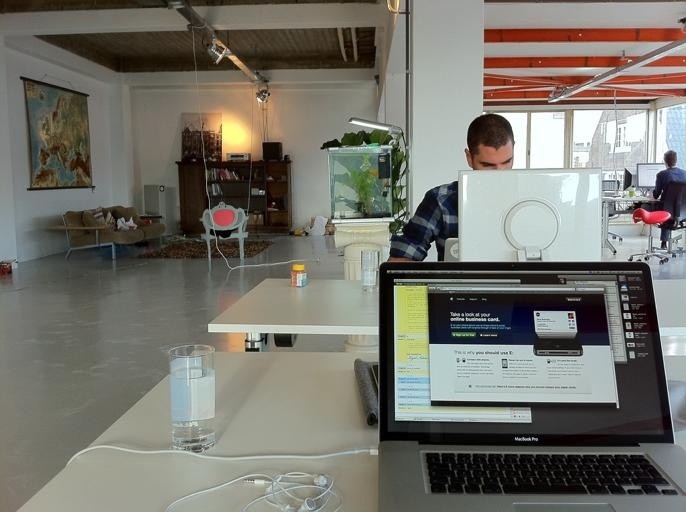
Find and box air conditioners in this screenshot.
[143,184,167,237]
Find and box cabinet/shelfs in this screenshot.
[175,161,293,238]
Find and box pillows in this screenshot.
[83,207,140,232]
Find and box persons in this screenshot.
[653,150,686,249]
[386,114,515,264]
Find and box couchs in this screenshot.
[46,205,166,260]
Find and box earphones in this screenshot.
[300,498,316,512]
[314,475,326,486]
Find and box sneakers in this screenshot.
[661,242,667,249]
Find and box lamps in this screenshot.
[348,117,403,145]
[166,0,270,103]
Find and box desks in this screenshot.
[601,191,660,255]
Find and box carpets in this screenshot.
[137,239,275,258]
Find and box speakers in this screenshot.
[262,142,282,159]
[443,238,458,261]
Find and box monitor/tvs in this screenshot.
[456,167,603,262]
[375,260,685,512]
[636,163,666,197]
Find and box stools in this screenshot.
[628,207,672,265]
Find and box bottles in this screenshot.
[290,264,308,288]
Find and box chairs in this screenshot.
[646,181,686,259]
[200,201,249,266]
[601,180,623,241]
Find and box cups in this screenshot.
[628,187,637,198]
[360,248,380,293]
[167,344,218,454]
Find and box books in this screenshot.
[206,168,239,181]
